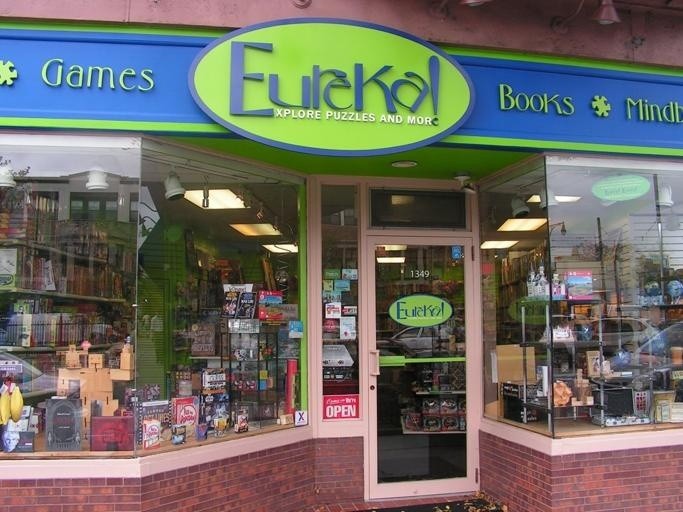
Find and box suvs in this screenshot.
[378,339,464,431]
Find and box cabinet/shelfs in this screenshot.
[0,235,134,400]
[171,241,682,437]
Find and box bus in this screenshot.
[0,286,131,380]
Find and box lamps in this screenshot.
[0,164,17,204]
[510,180,560,219]
[597,176,674,207]
[85,152,186,201]
[550,0,622,36]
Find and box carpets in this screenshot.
[352,497,505,512]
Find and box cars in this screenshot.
[627,277,682,304]
[0,328,8,344]
[91,323,120,338]
[479,318,682,403]
[0,350,80,406]
[385,320,464,352]
[320,324,354,355]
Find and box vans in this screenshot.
[6,313,92,344]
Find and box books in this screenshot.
[0,189,135,350]
